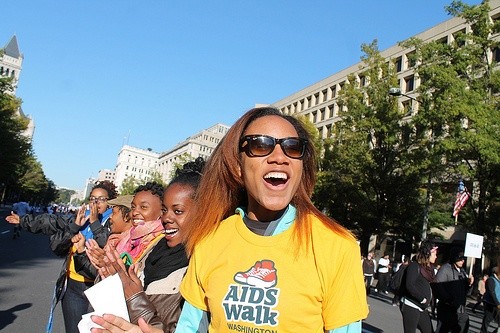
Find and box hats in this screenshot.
[449,251,467,263]
[106,194,136,208]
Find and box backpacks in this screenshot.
[387,261,421,297]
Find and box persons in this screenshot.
[392,254,410,306]
[89,106,369,332]
[472,274,488,314]
[399,239,439,333]
[480,267,500,333]
[85,181,166,284]
[362,253,374,296]
[375,253,392,294]
[45,204,79,213]
[98,156,208,332]
[368,251,377,286]
[72,194,135,278]
[430,251,474,333]
[6,181,120,333]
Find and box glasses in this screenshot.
[239,134,309,157]
[89,195,111,203]
